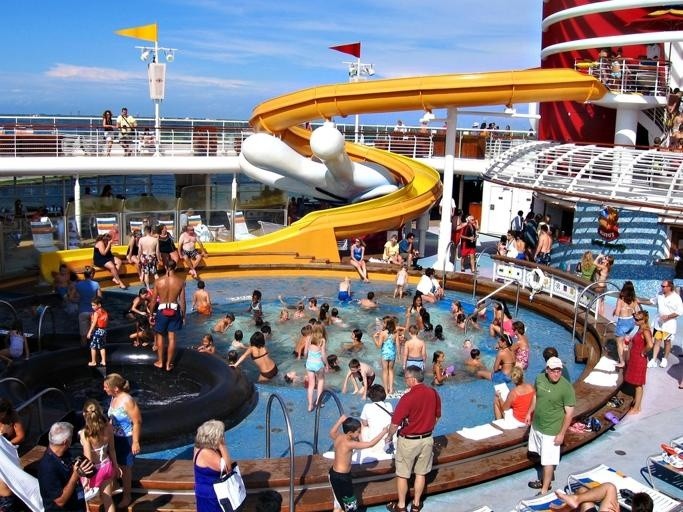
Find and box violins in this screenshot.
[39,68,606,283]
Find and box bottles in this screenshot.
[545,356,563,372]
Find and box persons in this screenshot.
[113,108,138,157]
[328,365,442,512]
[139,126,155,148]
[197,265,530,412]
[586,43,682,153]
[192,420,232,512]
[288,197,304,225]
[1,373,141,511]
[3,184,211,371]
[494,279,682,512]
[350,196,614,315]
[302,103,536,142]
[102,110,116,157]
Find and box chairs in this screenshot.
[647,357,670,369]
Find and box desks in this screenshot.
[342,41,373,142]
[132,19,178,155]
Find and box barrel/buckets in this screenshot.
[570,394,626,433]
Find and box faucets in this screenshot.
[526,478,553,489]
[385,498,423,512]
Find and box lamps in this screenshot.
[467,434,683,512]
[28,211,259,256]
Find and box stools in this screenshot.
[398,431,432,439]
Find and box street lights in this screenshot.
[214,456,248,511]
[575,262,582,273]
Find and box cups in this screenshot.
[632,312,645,322]
[660,284,670,289]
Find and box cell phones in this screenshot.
[74,454,97,478]
